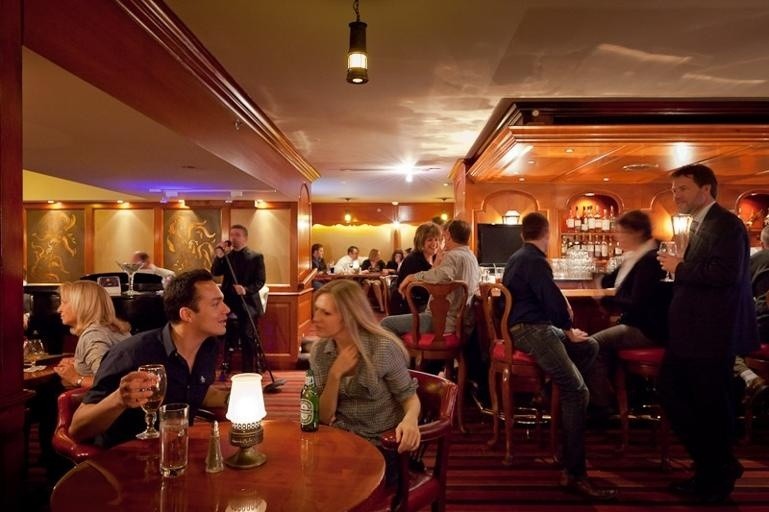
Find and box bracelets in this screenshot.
[76,373,84,386]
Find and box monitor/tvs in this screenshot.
[477,223,524,273]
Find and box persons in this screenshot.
[654,165,758,511]
[573,210,665,412]
[308,277,422,506]
[380,219,480,346]
[311,243,410,313]
[67,269,232,453]
[211,225,265,382]
[750,225,769,317]
[396,223,443,314]
[131,251,175,290]
[54,280,132,388]
[495,213,620,499]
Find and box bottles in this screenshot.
[298,369,321,432]
[205,419,223,475]
[206,475,225,511]
[298,435,322,502]
[557,202,624,260]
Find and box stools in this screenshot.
[610,344,677,462]
[736,339,769,450]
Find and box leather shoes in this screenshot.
[559,474,618,498]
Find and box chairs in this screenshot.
[52,381,229,477]
[475,279,564,469]
[395,271,477,442]
[363,368,465,512]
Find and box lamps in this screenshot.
[223,366,271,468]
[343,0,369,87]
[501,210,522,225]
[671,210,692,251]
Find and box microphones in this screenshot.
[217,241,232,249]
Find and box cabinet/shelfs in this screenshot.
[559,231,622,264]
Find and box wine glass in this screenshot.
[22,340,45,374]
[658,239,679,285]
[114,258,145,298]
[134,363,168,438]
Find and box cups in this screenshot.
[157,401,190,477]
[158,480,188,511]
[549,248,598,282]
[494,267,504,291]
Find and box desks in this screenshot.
[45,406,390,512]
[23,349,79,493]
[310,272,392,317]
